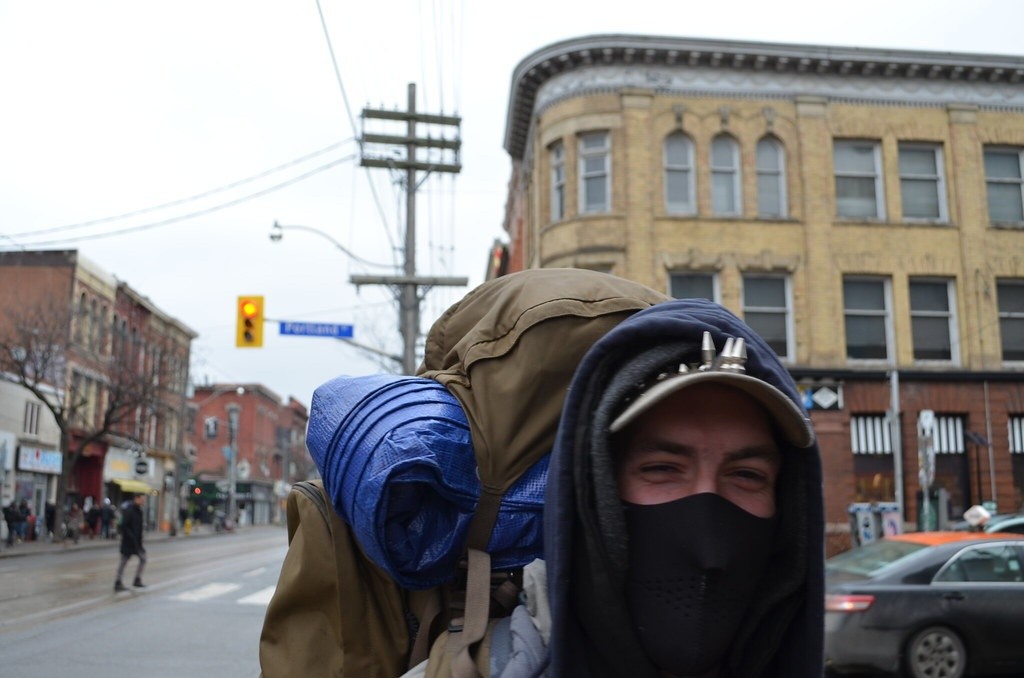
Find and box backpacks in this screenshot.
[257,261,679,678]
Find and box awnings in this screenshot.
[112,477,152,494]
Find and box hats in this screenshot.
[598,326,820,454]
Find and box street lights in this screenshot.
[269,220,417,376]
[168,385,245,540]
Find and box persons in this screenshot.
[100,497,117,539]
[18,500,31,539]
[46,499,55,533]
[115,496,148,590]
[86,502,100,539]
[3,500,21,548]
[67,502,84,544]
[400,297,826,678]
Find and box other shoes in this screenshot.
[112,582,131,593]
[133,580,146,588]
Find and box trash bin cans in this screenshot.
[915,487,939,533]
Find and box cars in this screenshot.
[824,505,1023,678]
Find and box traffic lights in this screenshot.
[189,484,217,496]
[236,295,263,349]
